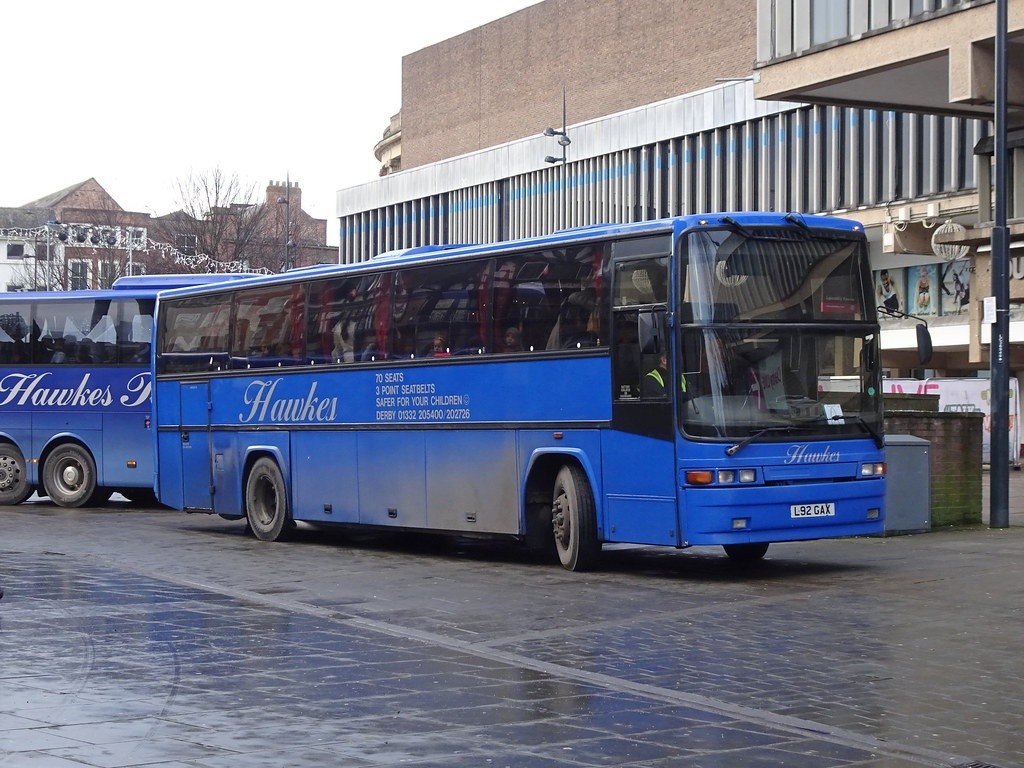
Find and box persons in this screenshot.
[952,273,966,315]
[914,266,933,316]
[172,322,523,354]
[644,346,689,404]
[11,334,149,365]
[877,269,903,313]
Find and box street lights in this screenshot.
[277,172,295,270]
[22,237,44,292]
[543,84,572,230]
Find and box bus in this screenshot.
[0,274,273,510]
[149,210,933,573]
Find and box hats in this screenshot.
[503,327,521,343]
